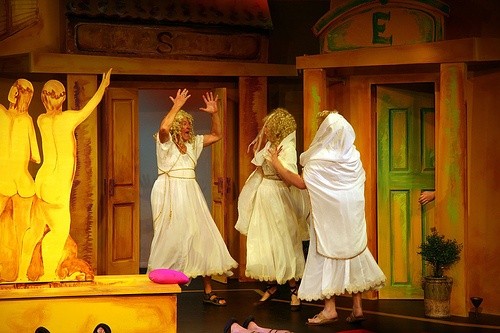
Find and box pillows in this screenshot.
[149,269,189,284]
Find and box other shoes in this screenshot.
[254,283,280,307]
[289,286,302,311]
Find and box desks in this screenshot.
[0,275,181,333]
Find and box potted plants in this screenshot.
[417,232,464,318]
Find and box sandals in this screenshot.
[202,291,227,305]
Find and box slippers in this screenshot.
[224,317,255,332]
[345,312,365,322]
[306,312,340,326]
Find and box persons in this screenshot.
[150,87,228,305]
[418,191,434,206]
[20,68,112,281]
[250,107,309,311]
[0,79,40,282]
[224,318,292,333]
[267,110,386,326]
